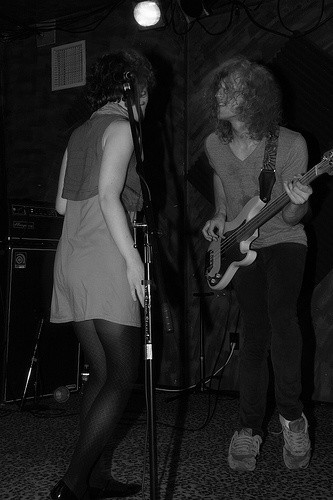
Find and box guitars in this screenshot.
[203,149,333,291]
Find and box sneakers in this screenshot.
[228,430,263,471]
[272,412,312,471]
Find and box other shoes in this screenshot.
[51,475,103,500]
[89,481,142,496]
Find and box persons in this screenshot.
[48,51,152,500]
[201,58,312,472]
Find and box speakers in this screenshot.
[0,246,82,403]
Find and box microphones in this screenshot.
[113,71,135,81]
